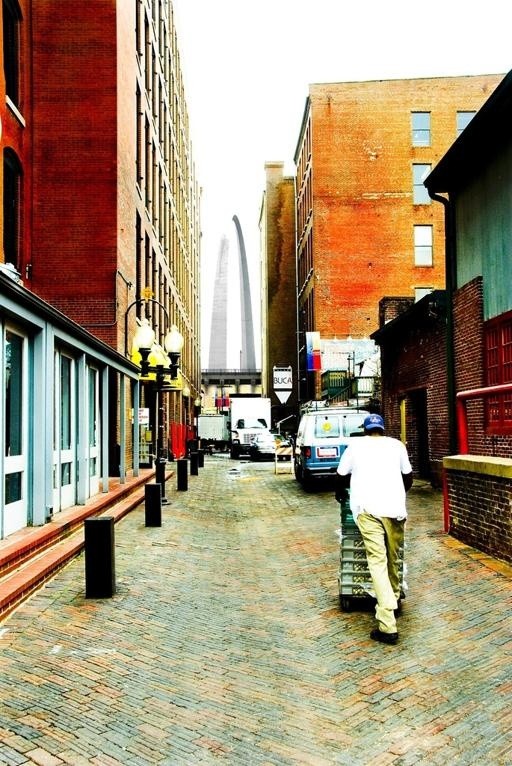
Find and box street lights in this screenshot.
[135,319,186,508]
[180,383,191,459]
[192,395,201,439]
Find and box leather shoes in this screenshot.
[394,602,403,618]
[369,628,399,645]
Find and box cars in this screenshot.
[251,434,291,462]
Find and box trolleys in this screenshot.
[336,487,408,615]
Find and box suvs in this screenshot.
[294,409,375,492]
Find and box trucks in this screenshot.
[228,397,271,461]
[198,414,230,454]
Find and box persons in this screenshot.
[336,413,414,645]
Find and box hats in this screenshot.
[363,414,384,431]
